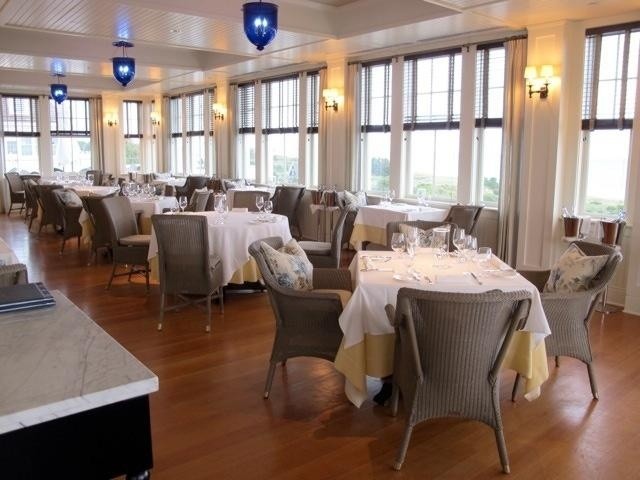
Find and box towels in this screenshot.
[576,217,604,243]
[361,256,392,271]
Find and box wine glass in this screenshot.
[271,175,277,190]
[406,225,423,259]
[114,180,143,200]
[431,228,450,264]
[179,196,187,212]
[464,237,477,263]
[214,195,227,225]
[256,196,264,218]
[390,233,405,260]
[424,195,432,211]
[264,201,273,222]
[478,247,492,279]
[143,183,149,198]
[246,176,252,191]
[170,201,178,212]
[453,229,465,262]
[149,187,156,200]
[87,174,95,194]
[80,180,87,195]
[388,190,397,206]
[417,189,427,207]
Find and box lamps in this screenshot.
[213,103,227,120]
[150,112,161,125]
[111,41,135,86]
[48,73,67,104]
[241,0,279,51]
[323,89,343,111]
[523,65,560,99]
[107,114,119,127]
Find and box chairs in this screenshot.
[175,175,210,201]
[510,241,623,402]
[184,190,210,211]
[20,175,41,214]
[298,207,350,269]
[151,214,226,332]
[81,192,117,266]
[385,221,458,252]
[233,191,270,212]
[102,197,152,293]
[442,205,484,235]
[384,287,532,475]
[4,172,26,216]
[269,186,304,224]
[335,191,371,250]
[51,189,84,256]
[33,185,64,235]
[248,236,354,399]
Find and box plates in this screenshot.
[249,218,268,225]
[494,270,520,279]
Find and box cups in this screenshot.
[394,273,429,283]
[242,207,249,217]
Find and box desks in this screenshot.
[66,186,119,197]
[147,211,293,303]
[0,290,159,480]
[350,203,448,253]
[78,195,180,260]
[333,248,552,409]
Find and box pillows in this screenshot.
[58,189,82,206]
[261,239,314,291]
[345,190,367,207]
[189,187,207,205]
[397,224,451,248]
[543,244,610,294]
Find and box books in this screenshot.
[1,283,57,314]
